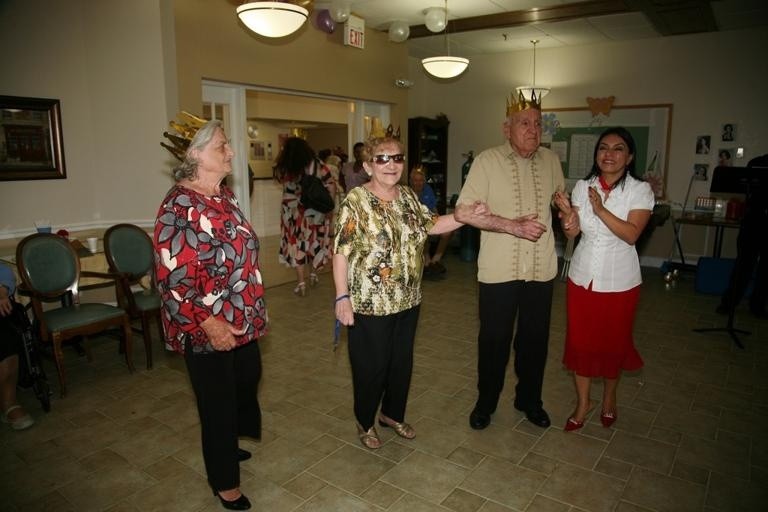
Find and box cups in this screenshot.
[86,236,98,253]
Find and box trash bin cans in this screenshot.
[660,261,698,296]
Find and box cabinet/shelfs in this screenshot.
[406,117,451,218]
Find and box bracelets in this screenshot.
[334,295,350,300]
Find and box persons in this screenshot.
[719,150,732,167]
[154,110,270,511]
[552,123,655,432]
[722,125,733,139]
[454,87,579,431]
[271,136,453,296]
[696,167,706,180]
[698,137,709,154]
[332,120,491,449]
[248,163,254,196]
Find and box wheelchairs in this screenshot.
[11,299,54,414]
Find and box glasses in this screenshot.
[375,154,404,163]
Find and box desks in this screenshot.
[0,238,123,358]
[658,211,740,277]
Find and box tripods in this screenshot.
[695,189,753,349]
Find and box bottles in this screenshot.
[726,197,740,220]
[696,197,715,209]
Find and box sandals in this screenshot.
[565,401,591,431]
[600,404,617,427]
[356,422,381,448]
[378,415,416,439]
[0,404,35,430]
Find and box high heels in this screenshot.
[309,273,319,286]
[294,282,306,297]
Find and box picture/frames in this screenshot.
[0,92,69,182]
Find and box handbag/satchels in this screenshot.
[300,173,334,213]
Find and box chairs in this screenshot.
[14,231,138,399]
[103,221,172,372]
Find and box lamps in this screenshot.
[514,40,551,103]
[226,0,318,41]
[420,0,471,80]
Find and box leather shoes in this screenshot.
[471,404,497,429]
[211,485,251,509]
[514,398,551,427]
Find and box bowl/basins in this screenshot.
[37,227,51,234]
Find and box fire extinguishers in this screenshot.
[461,151,474,188]
